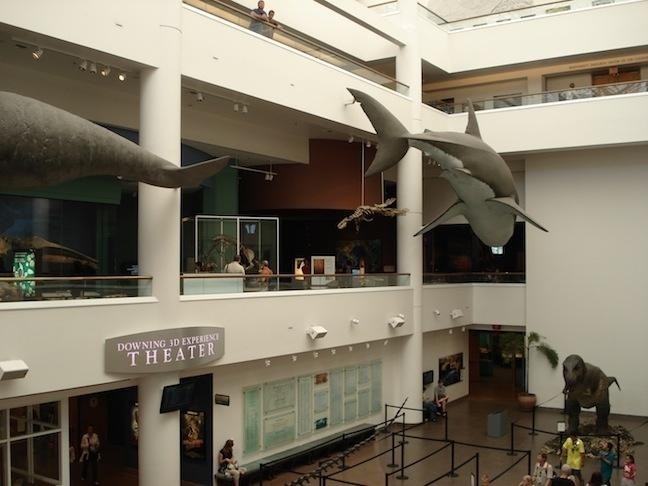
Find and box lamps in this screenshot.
[197,92,249,114]
[263,308,468,368]
[0,358,29,381]
[347,135,372,148]
[32,46,129,83]
[265,172,274,181]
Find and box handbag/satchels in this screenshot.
[545,464,557,476]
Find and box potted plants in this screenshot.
[499,331,558,412]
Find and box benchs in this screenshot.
[215,422,376,486]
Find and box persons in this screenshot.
[292,258,305,290]
[258,260,273,291]
[224,255,247,281]
[260,10,282,39]
[560,83,580,101]
[301,259,311,290]
[186,417,201,442]
[435,380,449,417]
[423,385,437,422]
[247,0,270,34]
[518,430,638,486]
[217,438,247,486]
[438,354,464,388]
[80,426,100,449]
[78,441,101,486]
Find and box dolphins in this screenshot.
[344,81,550,249]
